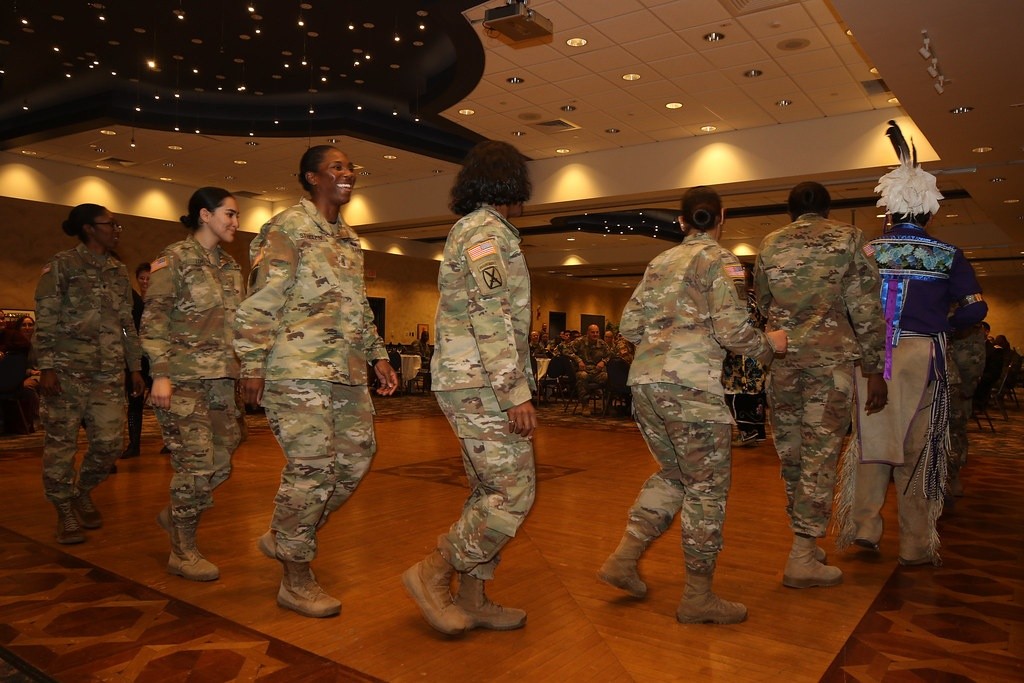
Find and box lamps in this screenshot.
[919,38,931,59]
[935,76,944,95]
[927,58,939,78]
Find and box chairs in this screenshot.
[0,352,32,434]
[365,340,1024,434]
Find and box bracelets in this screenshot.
[369,359,378,368]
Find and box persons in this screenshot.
[35,203,146,543]
[233,144,399,618]
[403,140,537,634]
[755,181,888,590]
[0,261,172,476]
[594,196,789,623]
[831,165,989,568]
[368,330,431,396]
[527,320,1022,497]
[140,186,246,582]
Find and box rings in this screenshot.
[508,421,514,424]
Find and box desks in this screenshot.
[400,354,422,396]
[535,358,551,380]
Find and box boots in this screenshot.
[167,520,219,583]
[277,560,340,617]
[596,530,651,599]
[401,546,473,634]
[947,477,964,497]
[261,524,281,558]
[454,569,526,629]
[52,499,86,543]
[156,502,177,536]
[782,533,843,589]
[675,564,748,625]
[71,487,103,529]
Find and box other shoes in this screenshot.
[120,446,140,459]
[582,403,591,416]
[856,538,880,554]
[901,550,936,566]
[732,433,756,446]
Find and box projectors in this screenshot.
[484,4,553,40]
